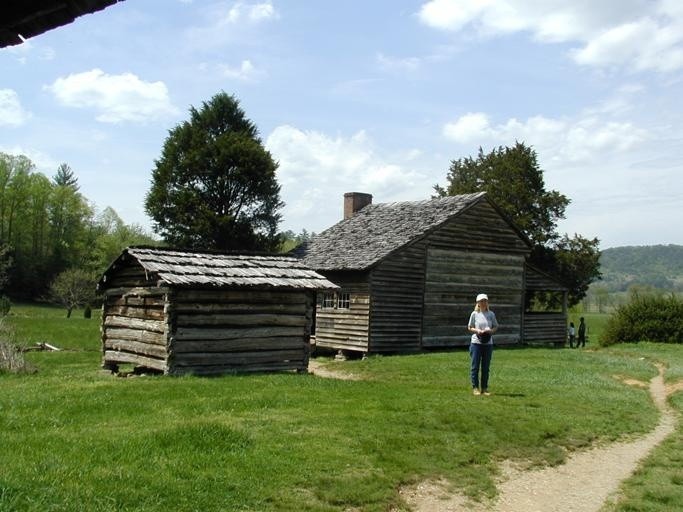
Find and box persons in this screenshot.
[568,322,576,348]
[576,317,585,347]
[467,294,499,395]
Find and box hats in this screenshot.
[476,293,488,302]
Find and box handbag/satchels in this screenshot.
[476,332,492,344]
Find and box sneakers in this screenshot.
[481,389,491,396]
[473,388,480,396]
